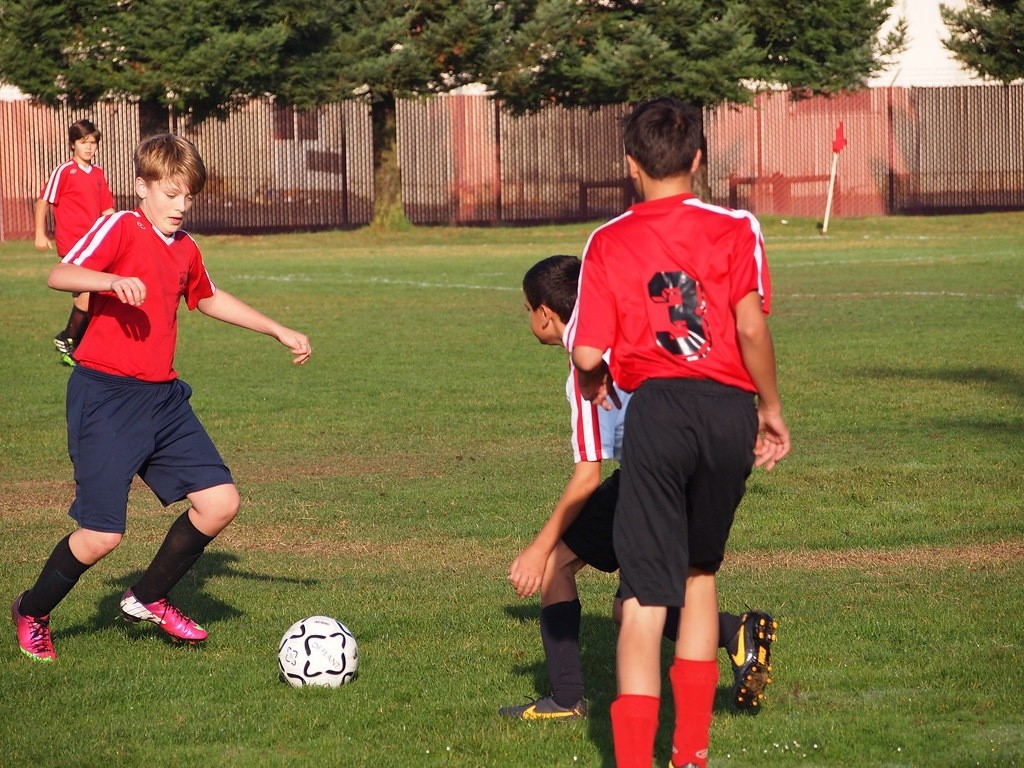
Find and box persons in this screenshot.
[571,100,792,768]
[495,255,777,722]
[12,134,312,666]
[35,119,115,366]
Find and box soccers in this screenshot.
[277,615,360,691]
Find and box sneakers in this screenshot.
[119,585,208,644]
[498,692,591,720]
[52,331,76,367]
[11,590,57,662]
[727,603,778,716]
